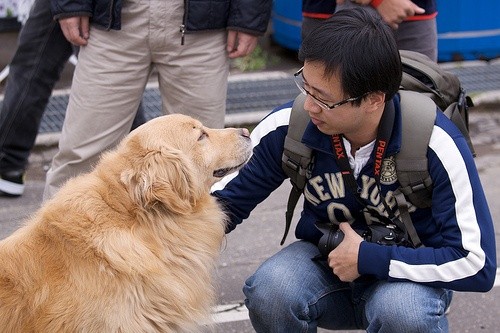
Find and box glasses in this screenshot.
[294,66,370,111]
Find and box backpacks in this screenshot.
[280,49,476,250]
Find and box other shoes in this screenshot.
[0,174,25,196]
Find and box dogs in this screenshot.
[0,114,253,332]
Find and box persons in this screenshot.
[0,0,149,197]
[44,1,272,202]
[300,0,439,67]
[209,8,497,333]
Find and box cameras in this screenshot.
[311,223,405,261]
